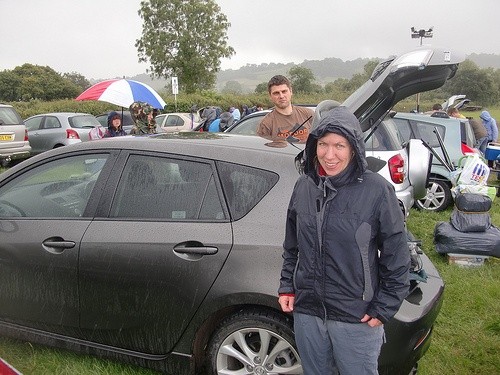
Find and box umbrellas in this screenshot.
[75,76,168,135]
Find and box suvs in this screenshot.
[0,103,32,167]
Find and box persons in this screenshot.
[102,112,127,138]
[256,73,316,141]
[433,103,498,152]
[129,101,158,135]
[264,141,288,149]
[276,106,411,375]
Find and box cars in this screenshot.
[93,111,200,135]
[22,113,106,158]
[1,133,445,374]
[222,46,482,221]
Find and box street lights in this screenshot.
[411,26,433,114]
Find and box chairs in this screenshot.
[118,161,172,218]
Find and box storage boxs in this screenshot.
[485,145,500,160]
[447,253,490,266]
